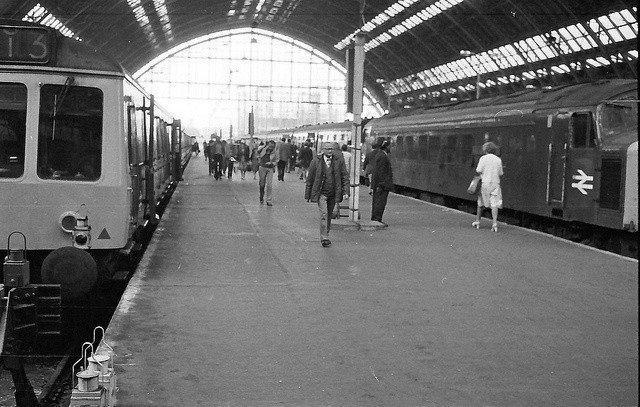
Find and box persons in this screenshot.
[304,142,350,246]
[370,139,392,227]
[257,141,280,206]
[194,140,199,156]
[471,141,505,233]
[287,139,316,180]
[363,143,376,193]
[341,145,351,173]
[203,133,256,180]
[277,138,290,181]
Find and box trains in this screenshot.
[1,19,195,357]
[242,76,639,257]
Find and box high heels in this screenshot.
[491,223,498,232]
[472,221,480,229]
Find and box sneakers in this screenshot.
[260,199,264,204]
[321,239,331,246]
[267,202,272,206]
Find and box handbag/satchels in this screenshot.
[466,176,481,194]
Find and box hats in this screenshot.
[322,143,335,149]
[376,137,390,149]
